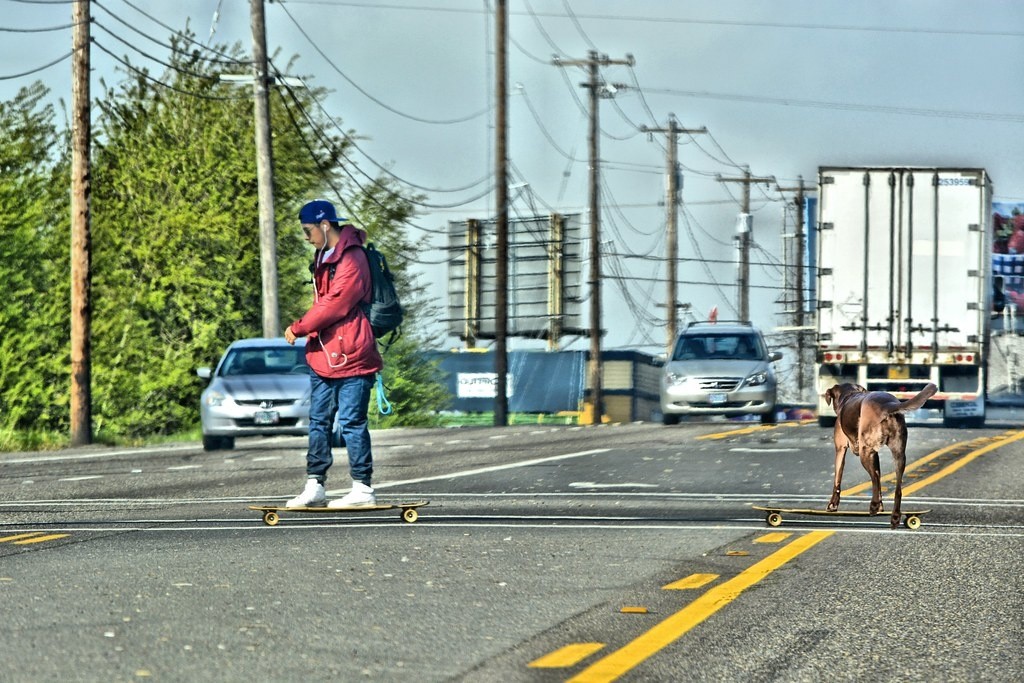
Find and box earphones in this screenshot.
[324,224,327,233]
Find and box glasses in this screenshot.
[304,225,318,235]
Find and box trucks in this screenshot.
[814,165,1005,428]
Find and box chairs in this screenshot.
[686,340,709,357]
[238,357,268,375]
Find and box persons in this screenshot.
[691,341,706,359]
[285,202,384,509]
[735,338,752,360]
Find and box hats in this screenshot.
[299,200,350,223]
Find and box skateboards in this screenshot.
[753,504,934,530]
[248,500,433,527]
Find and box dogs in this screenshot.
[825,383,938,527]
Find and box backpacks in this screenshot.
[327,242,403,352]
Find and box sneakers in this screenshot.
[328,481,377,509]
[286,478,326,508]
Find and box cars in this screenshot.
[197,339,346,451]
[653,320,782,426]
